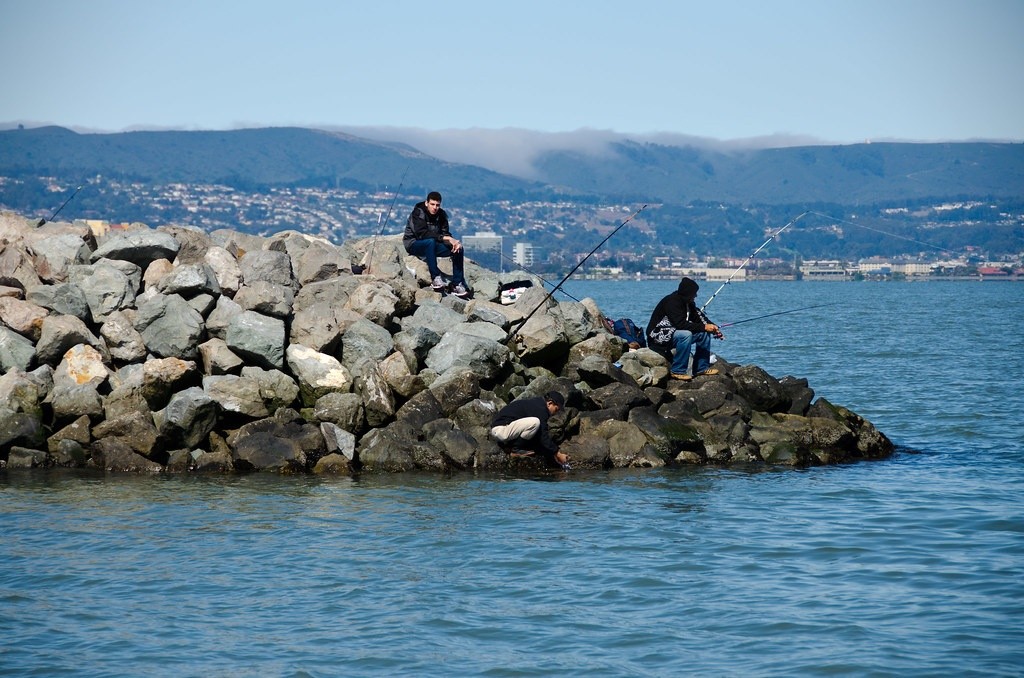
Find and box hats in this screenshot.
[547,391,566,412]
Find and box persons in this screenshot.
[645,277,724,381]
[403,191,469,298]
[492,390,571,465]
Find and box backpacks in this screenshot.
[613,318,646,349]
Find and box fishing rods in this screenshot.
[380,164,411,235]
[490,248,580,304]
[366,185,388,274]
[49,181,86,221]
[700,210,810,311]
[712,301,849,340]
[502,205,646,346]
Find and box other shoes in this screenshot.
[510,450,535,457]
[699,369,719,375]
[671,373,692,380]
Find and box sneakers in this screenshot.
[430,276,445,289]
[450,282,468,296]
